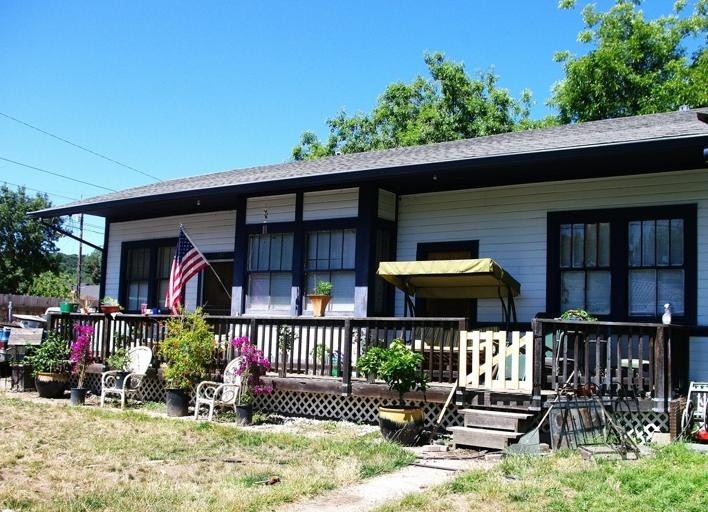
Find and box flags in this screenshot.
[163,227,210,315]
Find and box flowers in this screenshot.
[231,337,273,393]
[69,323,93,375]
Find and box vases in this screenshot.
[71,388,87,406]
[236,404,252,425]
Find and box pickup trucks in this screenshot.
[0,307,65,365]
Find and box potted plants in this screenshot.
[100,297,118,313]
[105,348,131,388]
[306,280,333,317]
[355,338,430,446]
[28,270,79,313]
[21,329,69,398]
[156,305,217,416]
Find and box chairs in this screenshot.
[194,356,248,422]
[100,346,152,410]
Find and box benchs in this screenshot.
[412,321,499,372]
